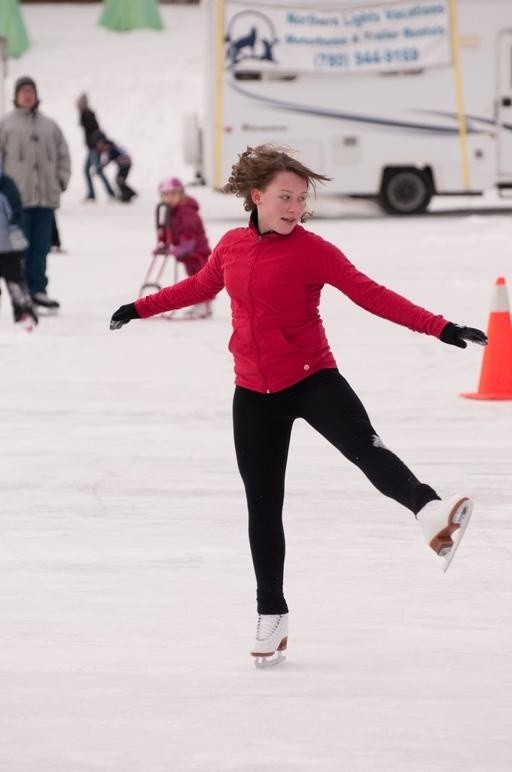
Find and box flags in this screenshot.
[110,144,488,672]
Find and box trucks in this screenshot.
[196,0,512,217]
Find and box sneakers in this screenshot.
[418,497,470,554]
[28,291,60,310]
[251,612,290,658]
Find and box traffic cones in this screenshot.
[459,273,512,402]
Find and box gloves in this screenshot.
[109,302,142,331]
[438,321,489,351]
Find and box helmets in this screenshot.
[159,176,185,197]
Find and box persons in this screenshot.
[1,76,72,315]
[74,94,119,201]
[94,133,137,202]
[0,149,40,331]
[154,178,214,316]
